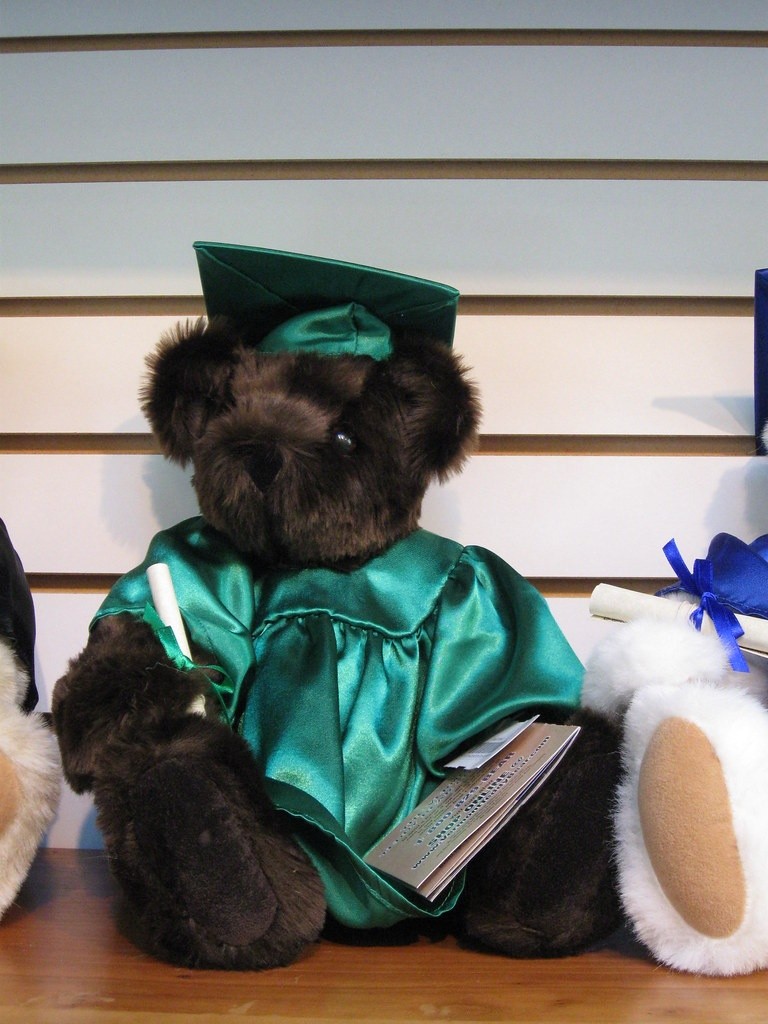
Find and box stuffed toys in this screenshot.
[51,240,631,972]
[582,531,768,976]
[0,516,65,917]
